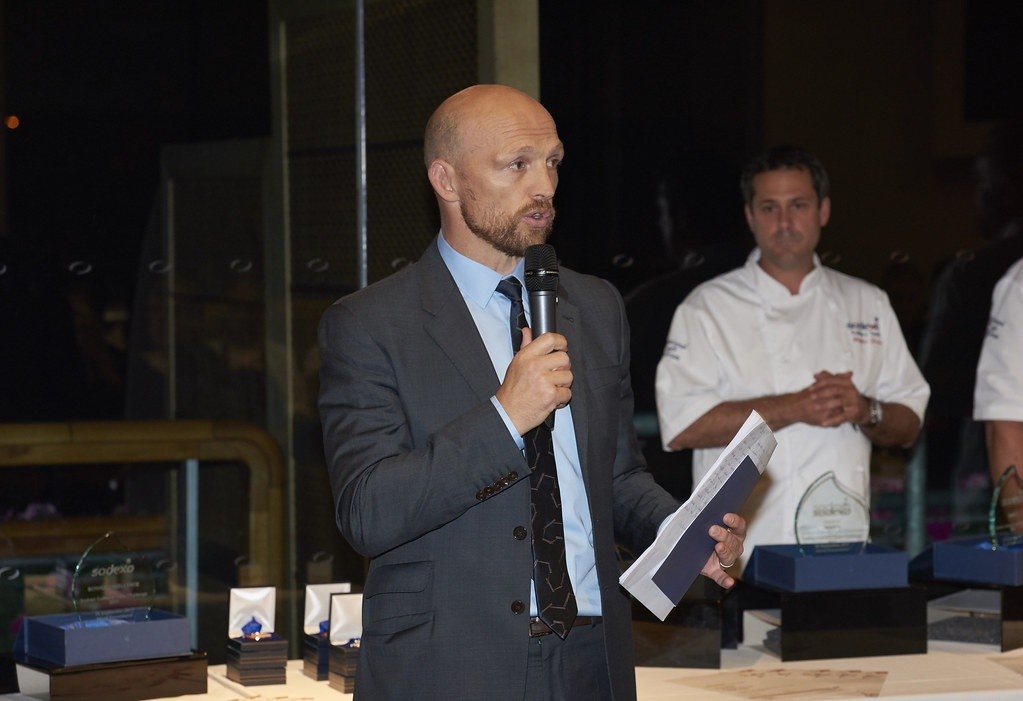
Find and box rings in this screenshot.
[720,559,736,568]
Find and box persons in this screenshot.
[318,84,746,701]
[973,259,1023,621]
[655,149,930,649]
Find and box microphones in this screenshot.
[524,243,561,431]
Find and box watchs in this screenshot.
[851,395,882,432]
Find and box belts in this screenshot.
[528,616,603,637]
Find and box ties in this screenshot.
[494,277,578,640]
